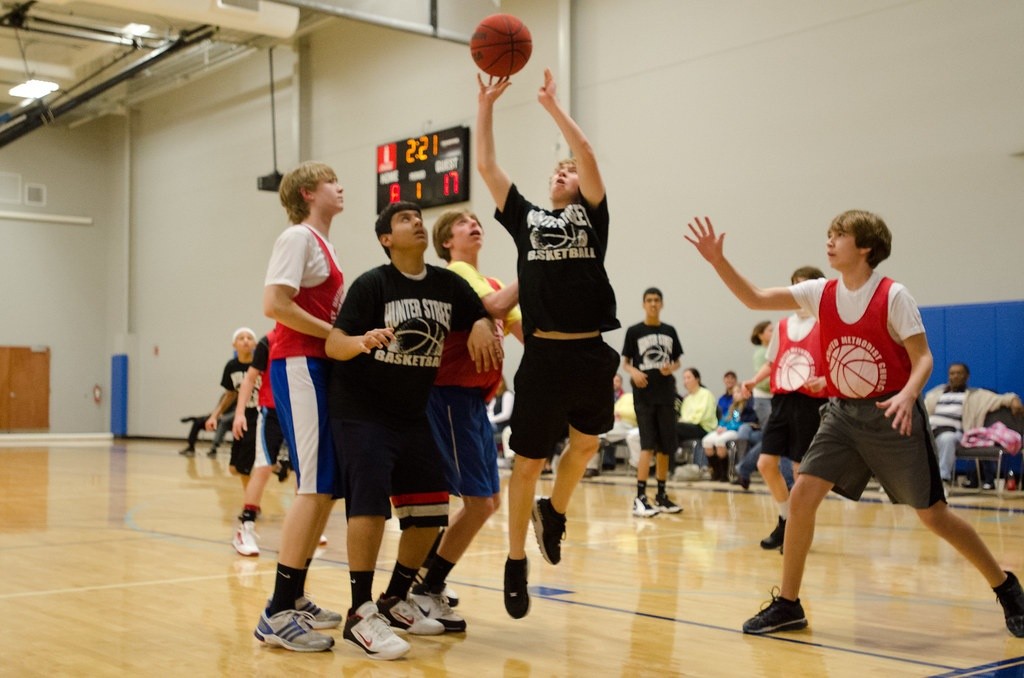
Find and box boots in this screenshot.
[705,452,730,482]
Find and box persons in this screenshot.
[178,390,237,457]
[324,201,524,660]
[622,288,685,518]
[879,362,1024,499]
[253,162,345,652]
[476,67,620,620]
[485,320,796,493]
[684,208,1024,640]
[741,266,829,554]
[206,327,327,556]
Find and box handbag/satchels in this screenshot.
[673,465,701,482]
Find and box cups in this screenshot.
[994,479,1005,490]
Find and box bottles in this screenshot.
[1007,469,1017,490]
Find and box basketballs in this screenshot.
[471,14,532,76]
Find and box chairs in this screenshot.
[728,397,773,482]
[951,407,1024,497]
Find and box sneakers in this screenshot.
[633,497,659,518]
[655,495,683,513]
[761,515,787,554]
[254,608,335,651]
[238,505,261,520]
[268,589,342,630]
[279,460,291,482]
[504,556,530,618]
[993,571,1024,638]
[319,535,327,544]
[376,593,445,634]
[530,497,567,565]
[413,567,458,606]
[232,520,259,556]
[342,601,410,661]
[407,582,466,632]
[742,585,808,634]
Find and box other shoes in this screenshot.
[943,480,951,498]
[208,449,217,456]
[180,447,194,454]
[540,469,554,480]
[583,470,599,477]
